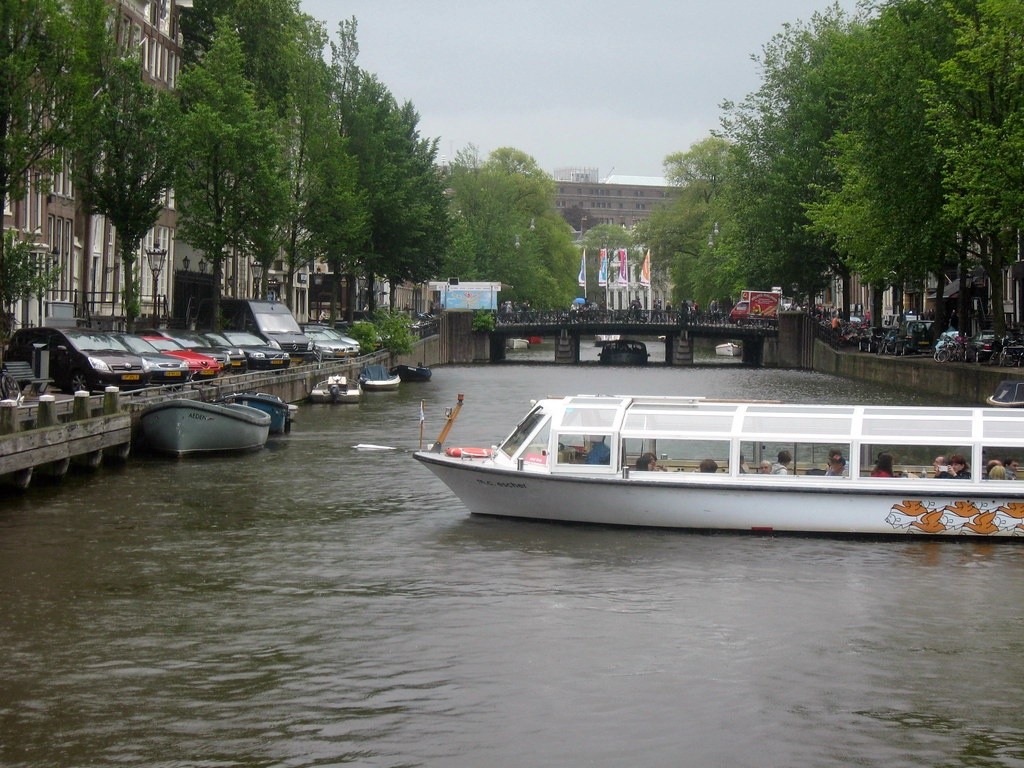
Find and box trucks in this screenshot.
[728,291,782,325]
[882,314,919,329]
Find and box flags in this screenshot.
[599,249,608,287]
[640,248,650,287]
[618,249,628,287]
[578,250,587,288]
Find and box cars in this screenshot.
[104,329,291,384]
[858,326,899,353]
[934,331,959,352]
[299,323,363,362]
[964,329,1013,363]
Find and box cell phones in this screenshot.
[939,466,948,472]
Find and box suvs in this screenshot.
[4,326,147,396]
[907,320,936,354]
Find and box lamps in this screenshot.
[49,246,60,262]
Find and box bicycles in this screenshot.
[820,318,872,346]
[934,335,1024,365]
[874,331,896,356]
[499,310,728,327]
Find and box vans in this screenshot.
[186,296,318,365]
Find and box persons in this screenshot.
[627,296,722,326]
[635,453,667,472]
[832,314,840,336]
[935,455,970,479]
[771,451,793,475]
[932,456,952,479]
[760,461,772,474]
[699,459,718,473]
[871,451,897,478]
[569,302,593,324]
[500,300,533,324]
[983,457,1020,480]
[824,447,849,476]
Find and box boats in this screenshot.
[593,334,621,348]
[657,335,667,343]
[142,398,276,457]
[360,367,402,392]
[986,380,1024,407]
[390,361,431,381]
[528,336,543,344]
[598,340,651,365]
[715,342,741,356]
[310,375,364,403]
[413,390,1024,539]
[221,391,300,434]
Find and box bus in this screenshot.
[307,272,380,322]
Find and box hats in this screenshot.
[828,454,846,466]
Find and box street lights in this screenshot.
[415,279,428,314]
[144,241,168,326]
[249,262,263,299]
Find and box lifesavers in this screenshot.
[445,446,491,457]
